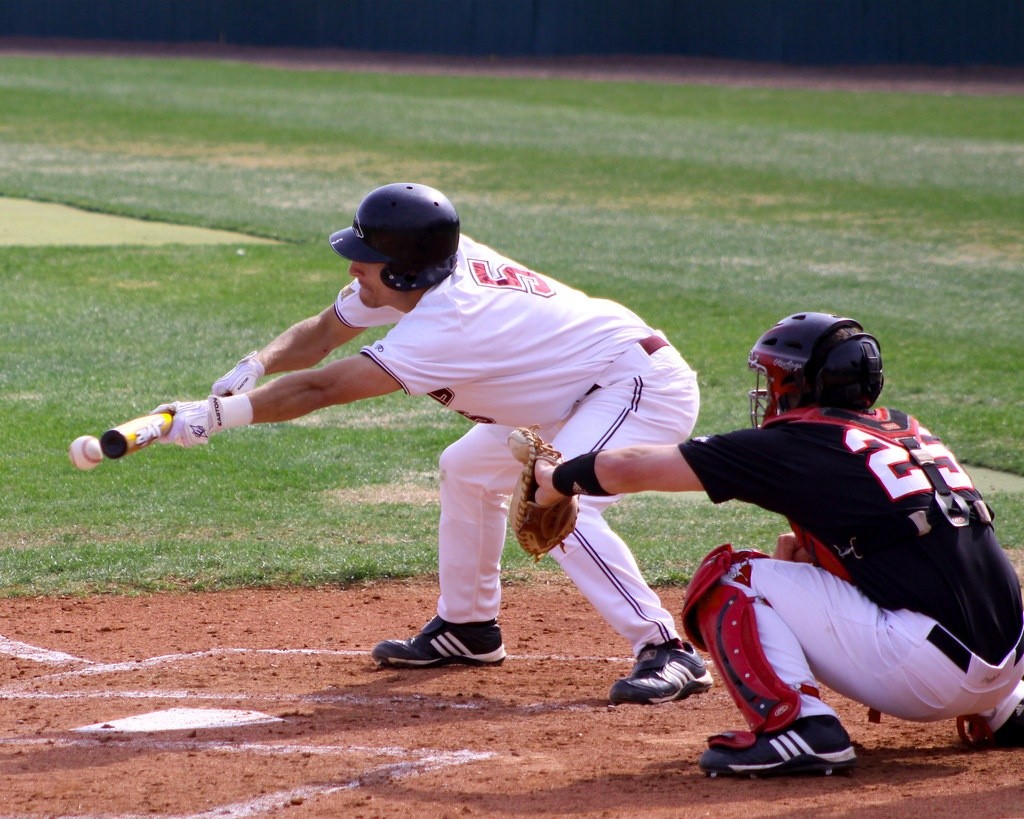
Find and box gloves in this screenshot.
[212,350,265,398]
[149,395,226,447]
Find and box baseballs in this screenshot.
[66,435,103,473]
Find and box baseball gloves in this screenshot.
[505,425,579,558]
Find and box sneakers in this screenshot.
[967,698,1024,748]
[609,641,713,705]
[700,715,857,779]
[372,615,506,668]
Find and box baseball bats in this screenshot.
[101,409,173,460]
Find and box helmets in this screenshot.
[748,312,884,431]
[329,182,460,292]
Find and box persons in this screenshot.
[151,182,714,704]
[534,311,1024,778]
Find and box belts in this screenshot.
[926,625,1024,674]
